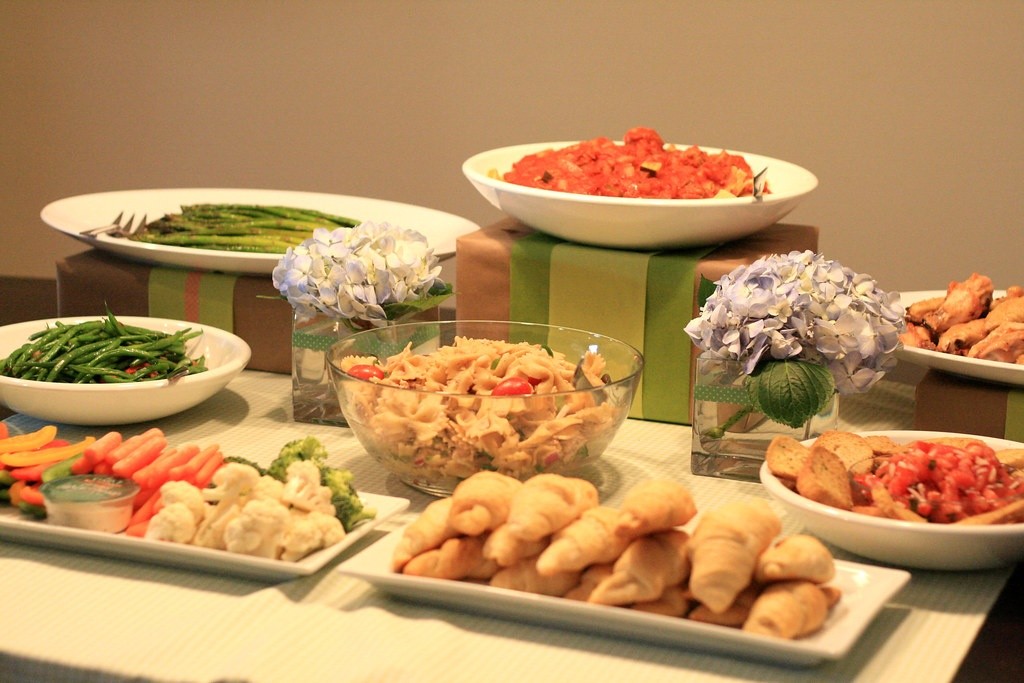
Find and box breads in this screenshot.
[766,429,1024,527]
[389,472,842,645]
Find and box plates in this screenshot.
[462,140,820,246]
[39,187,482,273]
[0,490,411,584]
[0,315,253,426]
[333,514,912,666]
[759,429,1024,571]
[889,288,1024,385]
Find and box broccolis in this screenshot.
[144,435,375,562]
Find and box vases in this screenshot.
[689,351,839,483]
[290,306,444,428]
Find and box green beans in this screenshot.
[0,302,209,384]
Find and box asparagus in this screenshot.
[123,203,361,255]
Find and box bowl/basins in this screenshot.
[324,318,646,497]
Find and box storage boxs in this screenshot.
[55,250,438,374]
[456,215,821,434]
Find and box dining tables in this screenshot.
[0,372,1024,683]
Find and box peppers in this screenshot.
[0,420,97,520]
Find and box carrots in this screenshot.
[64,428,226,538]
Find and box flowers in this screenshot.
[683,249,908,429]
[271,216,455,322]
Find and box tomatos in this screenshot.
[346,364,384,381]
[491,378,532,395]
